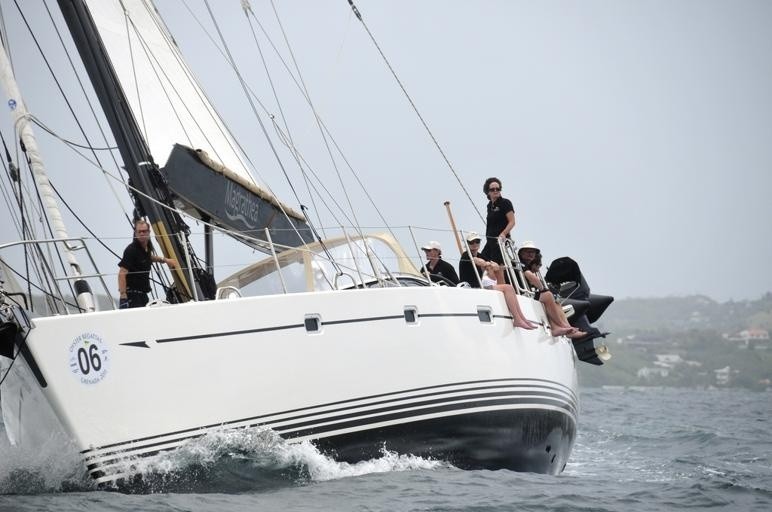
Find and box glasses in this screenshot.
[489,188,500,192]
[469,239,480,245]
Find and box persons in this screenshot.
[420,241,458,285]
[459,230,587,339]
[116,222,178,310]
[480,178,515,284]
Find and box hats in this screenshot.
[518,241,540,255]
[421,241,442,252]
[467,231,481,241]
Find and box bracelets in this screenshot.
[160,256,164,263]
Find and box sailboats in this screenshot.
[0,0,575,488]
